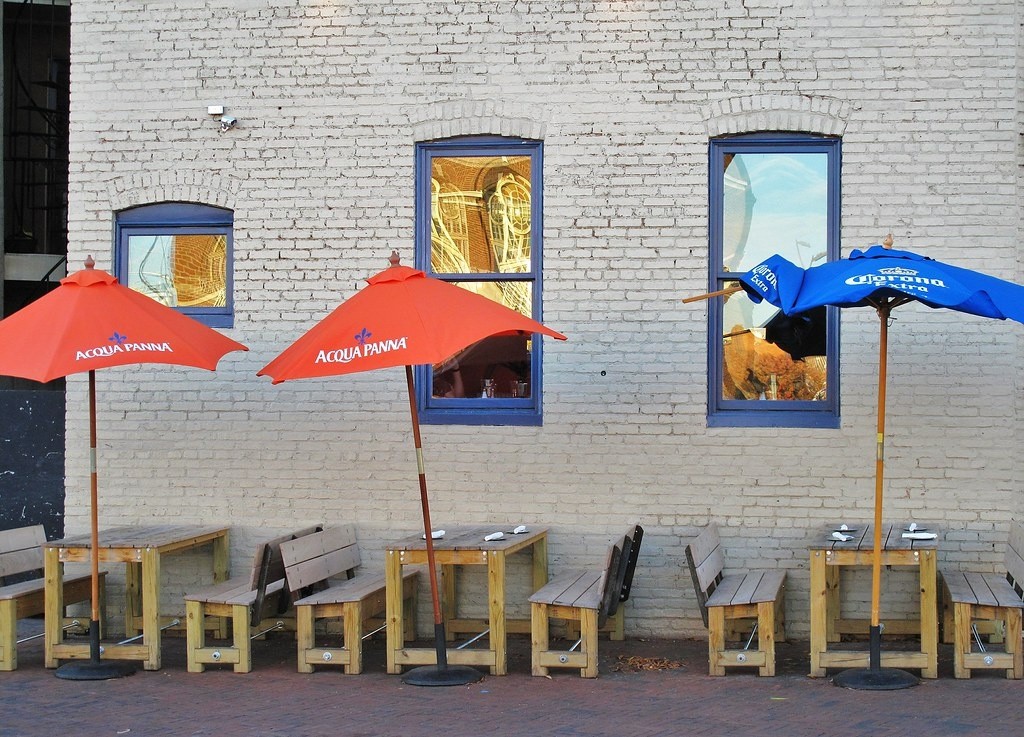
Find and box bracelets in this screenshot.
[452,369,460,372]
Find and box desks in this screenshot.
[808,522,941,679]
[40,525,231,671]
[385,525,550,674]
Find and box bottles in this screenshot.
[481,389,488,398]
[759,387,766,400]
[491,389,495,398]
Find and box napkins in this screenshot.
[832,532,848,542]
[514,525,526,534]
[901,532,937,540]
[484,532,504,542]
[423,529,445,539]
[909,522,918,532]
[840,524,850,530]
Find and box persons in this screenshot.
[434,355,464,398]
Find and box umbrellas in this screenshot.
[0,254,251,669]
[256,250,568,674]
[681,236,1024,672]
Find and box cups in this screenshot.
[481,378,495,398]
[510,380,527,398]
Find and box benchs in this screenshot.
[0,524,109,672]
[184,523,323,674]
[685,523,787,677]
[527,522,644,679]
[279,525,420,676]
[939,518,1024,680]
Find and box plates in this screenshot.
[828,535,854,541]
[507,530,529,534]
[833,528,858,532]
[420,536,443,540]
[910,538,934,540]
[903,527,927,531]
[484,537,506,541]
